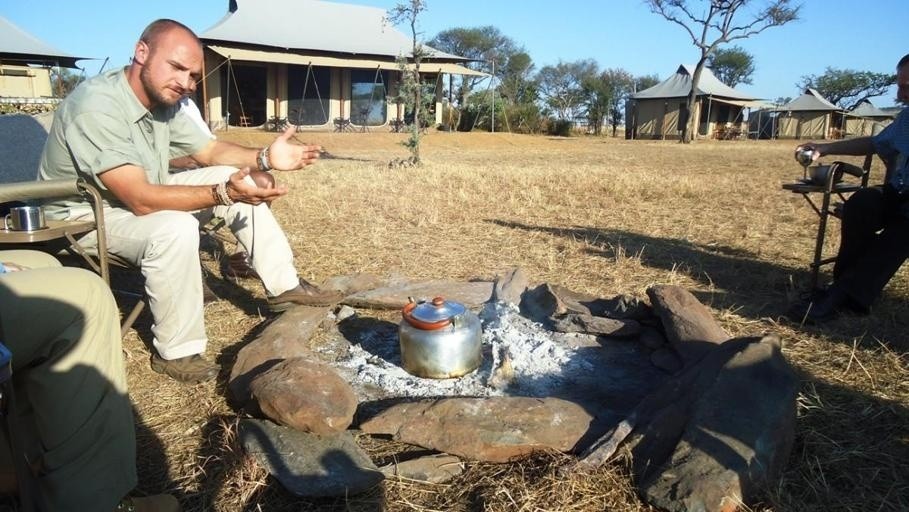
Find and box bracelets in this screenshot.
[808,164,843,182]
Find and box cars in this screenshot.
[4,206,50,233]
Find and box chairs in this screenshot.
[792,282,870,325]
[0,113,203,486]
[809,121,897,301]
[151,351,218,382]
[113,492,181,512]
[267,278,344,314]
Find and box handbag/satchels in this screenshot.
[214,182,235,206]
[255,145,271,173]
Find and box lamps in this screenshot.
[809,121,897,301]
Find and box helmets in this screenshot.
[795,177,845,186]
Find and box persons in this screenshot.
[1,248,186,511]
[792,53,909,327]
[31,16,348,385]
[165,44,279,306]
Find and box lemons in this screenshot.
[398,296,485,380]
